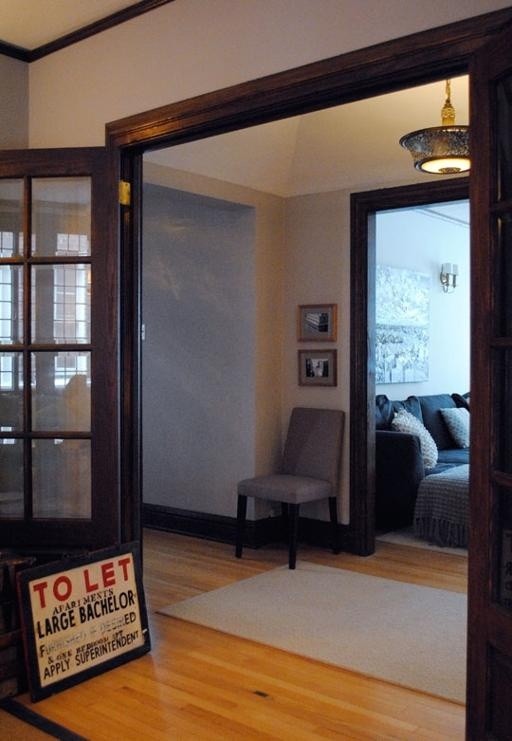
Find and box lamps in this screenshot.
[397,77,474,177]
[439,262,458,293]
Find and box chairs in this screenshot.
[231,407,347,575]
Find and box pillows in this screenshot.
[437,406,470,448]
[390,405,440,471]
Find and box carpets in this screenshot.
[153,560,467,706]
[373,524,468,557]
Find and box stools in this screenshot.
[411,464,470,551]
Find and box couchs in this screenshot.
[375,389,471,528]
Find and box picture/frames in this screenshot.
[297,349,338,388]
[296,302,339,345]
